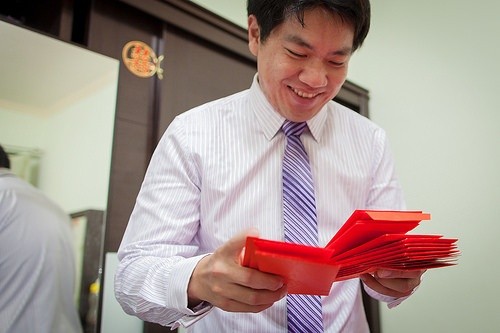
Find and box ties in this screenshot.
[283,118,325,333]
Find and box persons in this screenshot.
[0,144,83,333]
[114,1,427,333]
